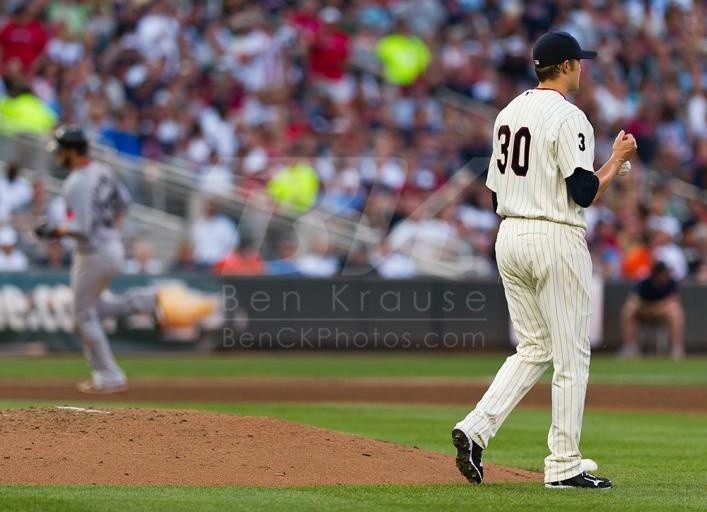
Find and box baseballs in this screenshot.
[616,160,631,176]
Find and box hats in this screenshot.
[532,29,598,70]
[42,122,89,157]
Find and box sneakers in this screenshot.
[450,426,484,486]
[74,375,128,395]
[542,470,613,491]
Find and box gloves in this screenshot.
[30,221,60,242]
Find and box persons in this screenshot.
[449,29,639,490]
[32,124,132,392]
[616,260,687,363]
